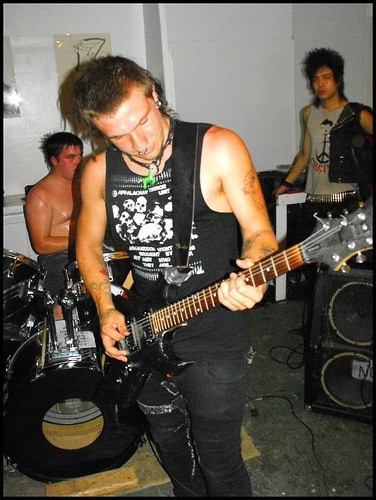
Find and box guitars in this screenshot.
[74,199,375,418]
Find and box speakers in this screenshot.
[305,261,375,423]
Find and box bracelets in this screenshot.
[282,179,293,188]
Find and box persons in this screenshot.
[71,57,280,496]
[273,47,373,233]
[23,132,84,339]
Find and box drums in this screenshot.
[69,251,129,296]
[3,250,39,307]
[0,319,147,483]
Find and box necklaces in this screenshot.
[123,118,176,189]
